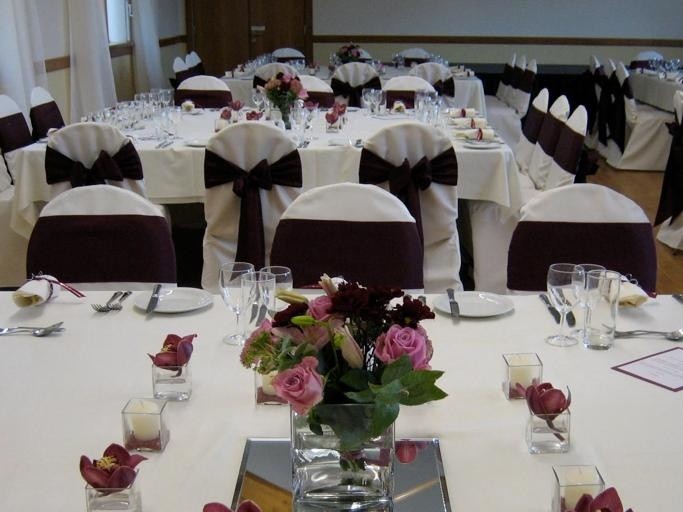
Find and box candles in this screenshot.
[507,355,533,391]
[565,466,598,500]
[230,110,238,122]
[129,398,162,443]
[215,118,227,130]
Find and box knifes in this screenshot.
[539,292,562,324]
[446,288,459,319]
[145,284,162,314]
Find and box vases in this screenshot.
[291,404,393,512]
[84,482,141,510]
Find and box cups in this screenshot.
[362,88,374,119]
[650,55,681,81]
[584,264,605,269]
[84,468,141,512]
[551,464,605,512]
[114,87,172,132]
[375,90,384,115]
[546,263,584,349]
[241,271,275,332]
[426,89,440,125]
[295,58,305,77]
[325,119,337,134]
[253,92,263,112]
[258,266,293,313]
[290,107,305,146]
[217,261,253,347]
[415,90,426,121]
[120,397,170,452]
[585,270,621,351]
[501,352,543,400]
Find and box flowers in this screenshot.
[220,110,230,125]
[79,445,147,493]
[230,100,243,117]
[521,382,571,446]
[303,100,319,114]
[324,109,337,127]
[147,333,196,379]
[238,272,451,512]
[246,111,263,124]
[337,41,360,63]
[334,102,346,120]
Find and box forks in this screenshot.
[90,291,122,312]
[107,291,131,312]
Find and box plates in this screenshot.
[433,290,515,318]
[461,142,500,149]
[347,106,358,112]
[132,287,213,314]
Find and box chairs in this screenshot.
[379,77,438,111]
[1,94,35,184]
[398,48,432,67]
[488,59,537,118]
[653,91,683,253]
[273,48,306,66]
[596,59,617,159]
[582,55,602,151]
[606,60,676,173]
[407,63,457,107]
[200,123,302,290]
[192,51,209,74]
[251,64,299,98]
[27,185,180,292]
[484,52,516,102]
[29,87,65,143]
[330,63,379,107]
[470,106,588,291]
[173,77,233,107]
[169,58,187,83]
[355,49,372,63]
[512,88,549,172]
[43,122,150,201]
[186,53,197,77]
[269,182,423,291]
[517,96,568,188]
[505,183,657,297]
[359,120,464,294]
[1,155,29,291]
[630,51,665,71]
[295,76,334,110]
[486,55,526,108]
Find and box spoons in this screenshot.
[0,322,64,338]
[622,329,682,341]
